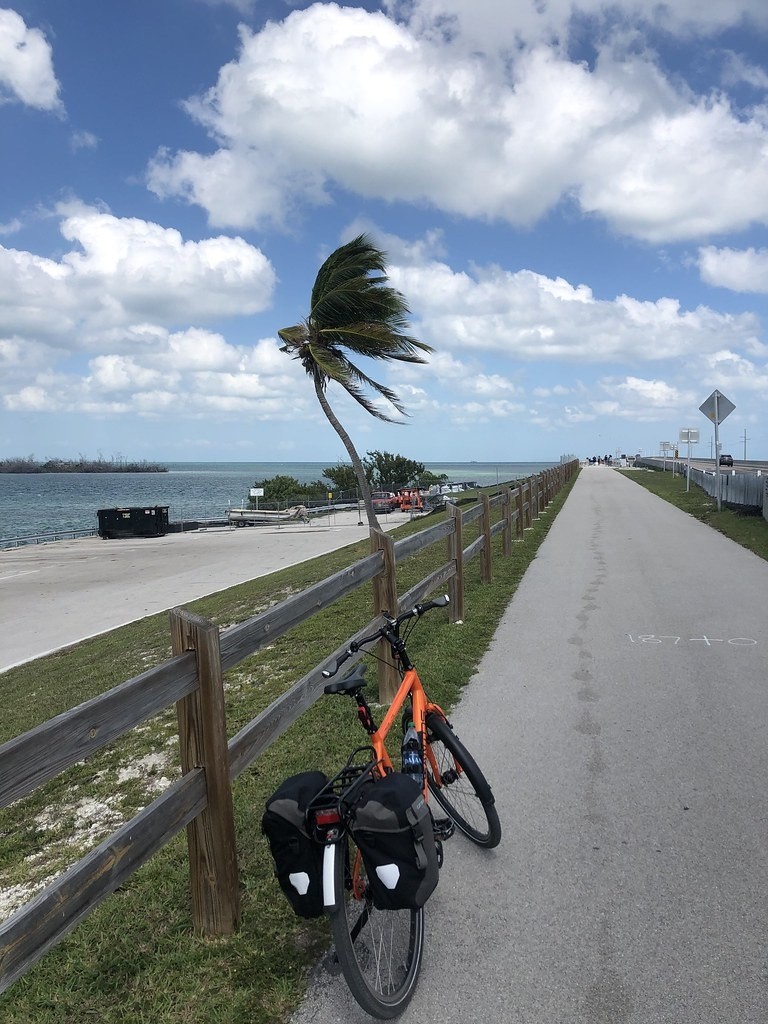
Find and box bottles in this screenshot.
[402,721,423,786]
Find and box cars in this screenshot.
[370,492,397,514]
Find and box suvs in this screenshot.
[719,455,734,467]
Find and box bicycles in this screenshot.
[303,593,504,1024]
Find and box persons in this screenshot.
[586,455,613,467]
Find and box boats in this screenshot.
[228,509,299,521]
